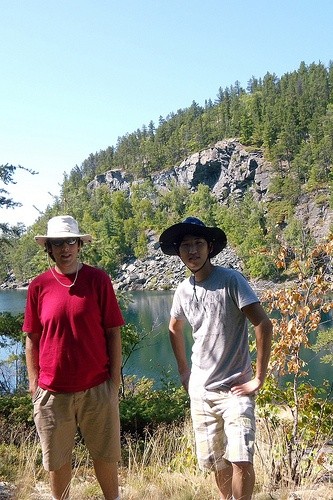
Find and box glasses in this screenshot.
[50,238,79,246]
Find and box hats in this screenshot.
[159,217,227,258]
[34,216,92,247]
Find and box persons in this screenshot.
[159,217,273,500]
[22,216,124,500]
